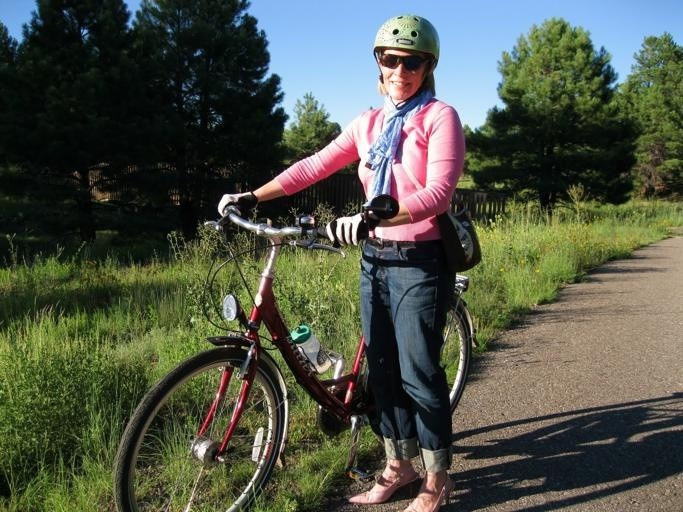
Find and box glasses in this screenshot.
[378,53,434,71]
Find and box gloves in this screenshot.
[326,210,381,247]
[216,190,257,222]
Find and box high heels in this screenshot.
[348,472,455,512]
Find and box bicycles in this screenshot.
[105,203,481,512]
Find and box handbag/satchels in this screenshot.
[451,205,482,271]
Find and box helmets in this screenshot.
[372,15,441,60]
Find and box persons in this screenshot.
[216,14,466,511]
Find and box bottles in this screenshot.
[290,324,332,374]
